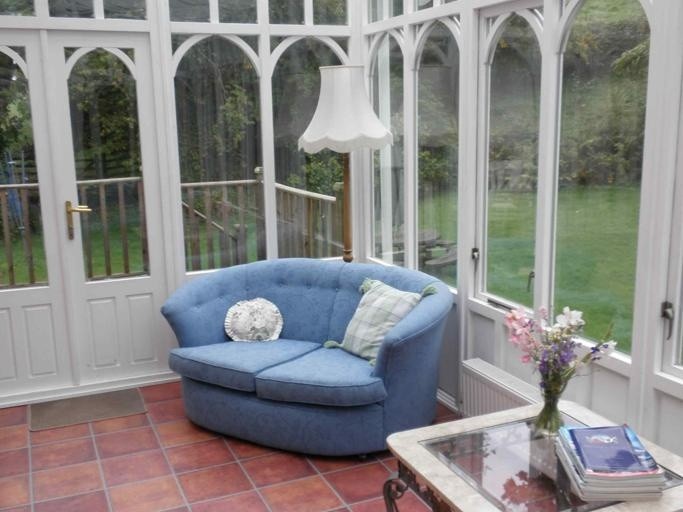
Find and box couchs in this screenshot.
[161,259,455,458]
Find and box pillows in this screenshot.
[225,297,283,343]
[325,277,439,365]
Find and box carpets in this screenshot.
[26,385,148,434]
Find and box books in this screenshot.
[551,421,668,506]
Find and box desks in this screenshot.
[385,395,683,511]
[373,228,441,264]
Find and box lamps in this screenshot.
[301,60,396,262]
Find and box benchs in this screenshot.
[424,247,458,267]
[427,239,457,247]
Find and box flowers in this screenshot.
[502,309,617,423]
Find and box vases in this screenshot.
[530,380,569,436]
